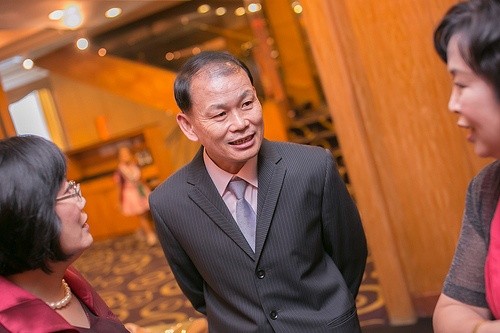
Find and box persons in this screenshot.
[0,134,132,333]
[433,0,500,333]
[148,51,367,333]
[113,146,156,247]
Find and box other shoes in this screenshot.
[146,233,157,247]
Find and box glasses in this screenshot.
[56,180,82,201]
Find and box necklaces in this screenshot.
[44,278,72,309]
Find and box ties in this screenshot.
[228,179,257,253]
